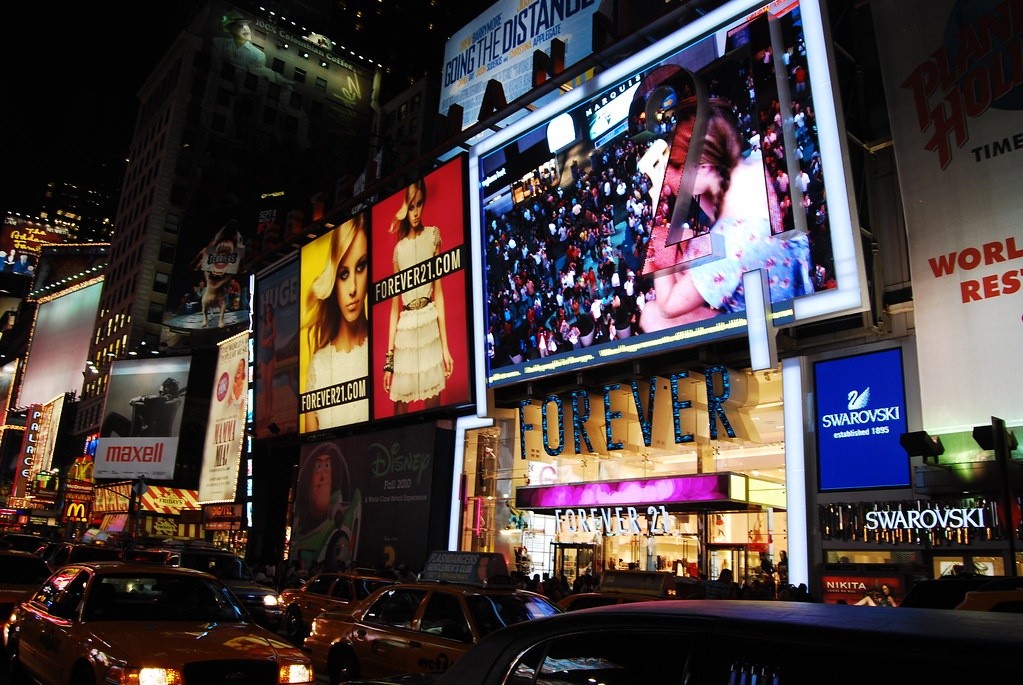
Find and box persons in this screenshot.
[213,10,290,80]
[480,20,839,372]
[179,262,249,324]
[380,177,454,410]
[259,303,277,421]
[304,214,374,431]
[99,375,180,438]
[226,553,905,607]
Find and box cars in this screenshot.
[1,534,684,641]
[1,548,316,685]
[345,600,1022,685]
